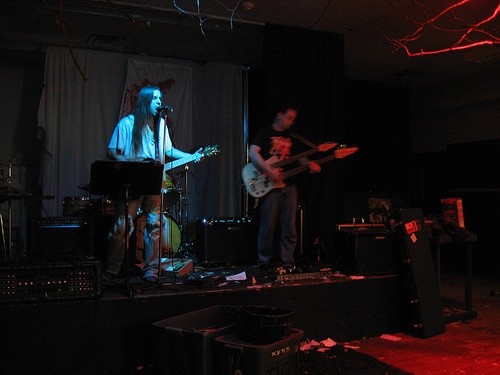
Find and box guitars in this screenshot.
[242,142,359,197]
[109,145,219,205]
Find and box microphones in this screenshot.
[156,106,172,112]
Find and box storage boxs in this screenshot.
[152,305,303,374]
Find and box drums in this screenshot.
[159,173,180,210]
[62,196,96,218]
[133,213,180,265]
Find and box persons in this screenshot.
[248,106,320,275]
[106,85,206,286]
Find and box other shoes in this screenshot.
[112,272,121,280]
[145,270,158,282]
[285,264,302,273]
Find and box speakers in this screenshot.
[25,216,93,259]
[192,217,258,265]
[335,233,403,275]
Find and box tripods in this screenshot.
[89,159,164,297]
